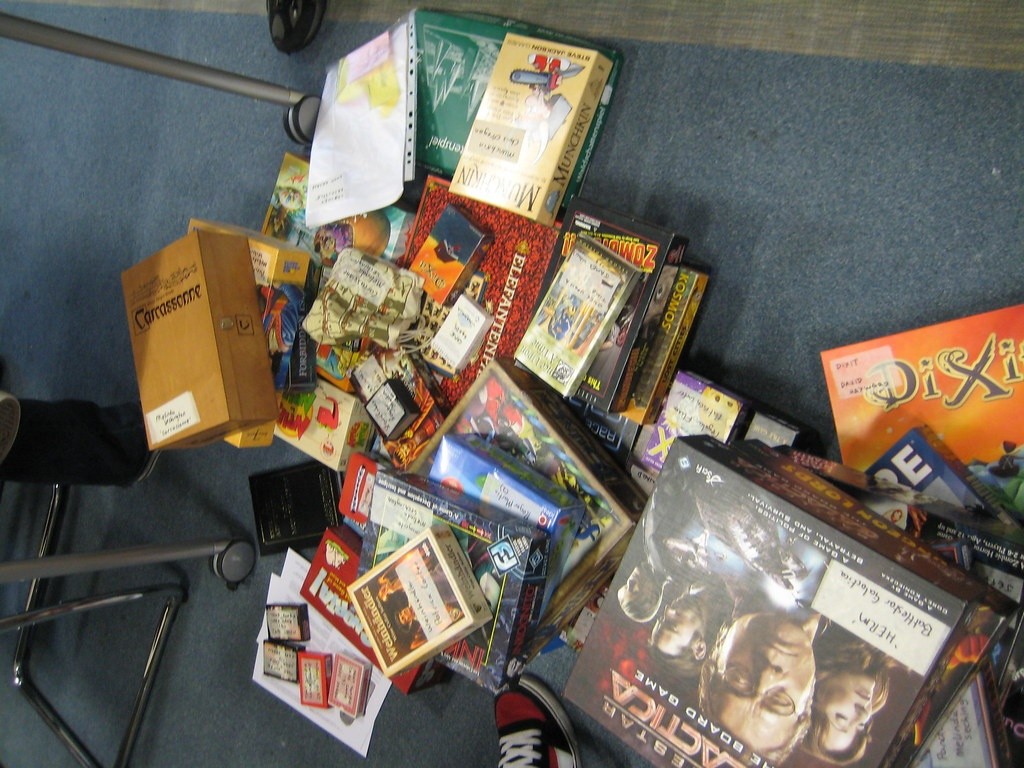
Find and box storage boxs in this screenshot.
[118,7,1024,768]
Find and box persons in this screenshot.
[493,674,581,768]
[379,547,464,651]
[618,456,901,767]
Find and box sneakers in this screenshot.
[493,674,584,768]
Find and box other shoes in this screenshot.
[265,0,327,53]
